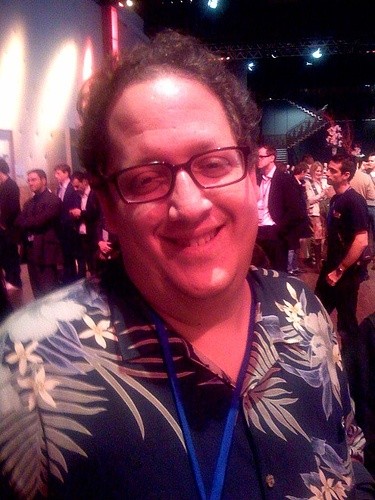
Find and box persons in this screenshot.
[0,156,22,290]
[305,160,331,274]
[289,162,314,275]
[16,168,63,298]
[345,155,375,201]
[0,27,375,500]
[96,216,120,259]
[358,152,375,268]
[314,152,370,333]
[341,308,375,478]
[53,163,75,285]
[252,145,291,273]
[276,155,329,268]
[70,170,94,279]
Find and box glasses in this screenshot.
[102,146,250,204]
[257,154,274,159]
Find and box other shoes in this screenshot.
[288,272,298,276]
[293,268,304,273]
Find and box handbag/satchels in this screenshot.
[320,201,328,219]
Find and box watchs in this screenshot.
[335,266,345,277]
